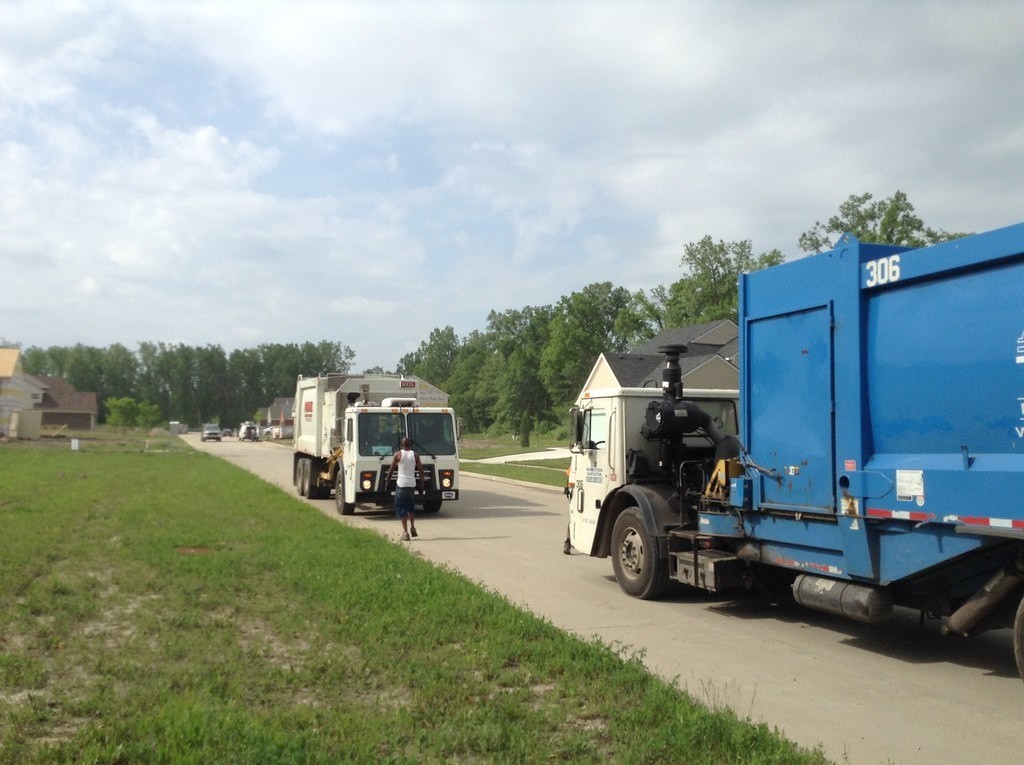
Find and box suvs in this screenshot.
[201,423,221,442]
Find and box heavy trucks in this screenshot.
[290,370,464,515]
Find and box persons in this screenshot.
[251,429,255,441]
[384,438,424,542]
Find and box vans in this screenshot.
[239,422,260,442]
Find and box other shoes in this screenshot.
[400,533,410,541]
[564,541,571,554]
[411,528,418,536]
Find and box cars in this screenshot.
[263,425,274,438]
[222,429,232,437]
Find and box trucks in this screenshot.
[562,217,1024,678]
